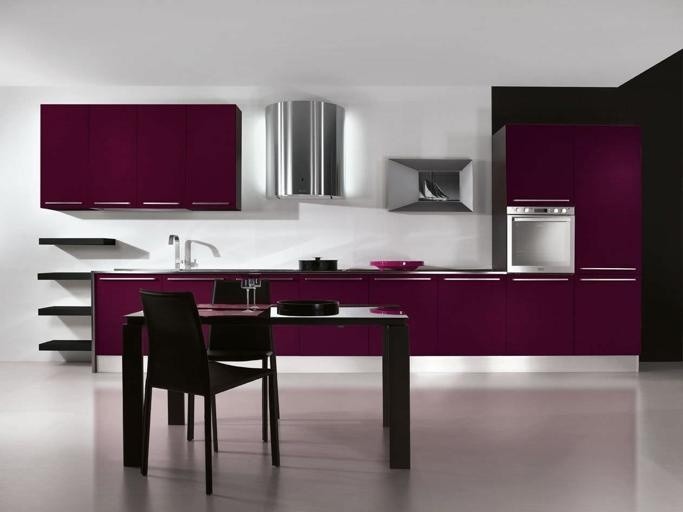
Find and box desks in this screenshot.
[120,308,413,471]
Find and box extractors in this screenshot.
[264,98,345,201]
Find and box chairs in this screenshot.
[136,288,280,498]
[197,277,281,442]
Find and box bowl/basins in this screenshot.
[370,260,425,271]
[277,300,339,315]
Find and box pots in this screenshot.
[299,257,338,273]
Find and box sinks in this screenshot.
[113,267,183,272]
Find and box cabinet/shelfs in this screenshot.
[268,278,369,356]
[165,278,215,343]
[370,277,508,355]
[490,122,571,271]
[569,124,641,272]
[33,239,117,351]
[94,275,164,356]
[38,103,241,213]
[509,277,640,355]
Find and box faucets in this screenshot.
[184,239,220,270]
[168,234,185,269]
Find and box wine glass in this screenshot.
[238,279,261,313]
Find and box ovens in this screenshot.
[506,205,577,275]
